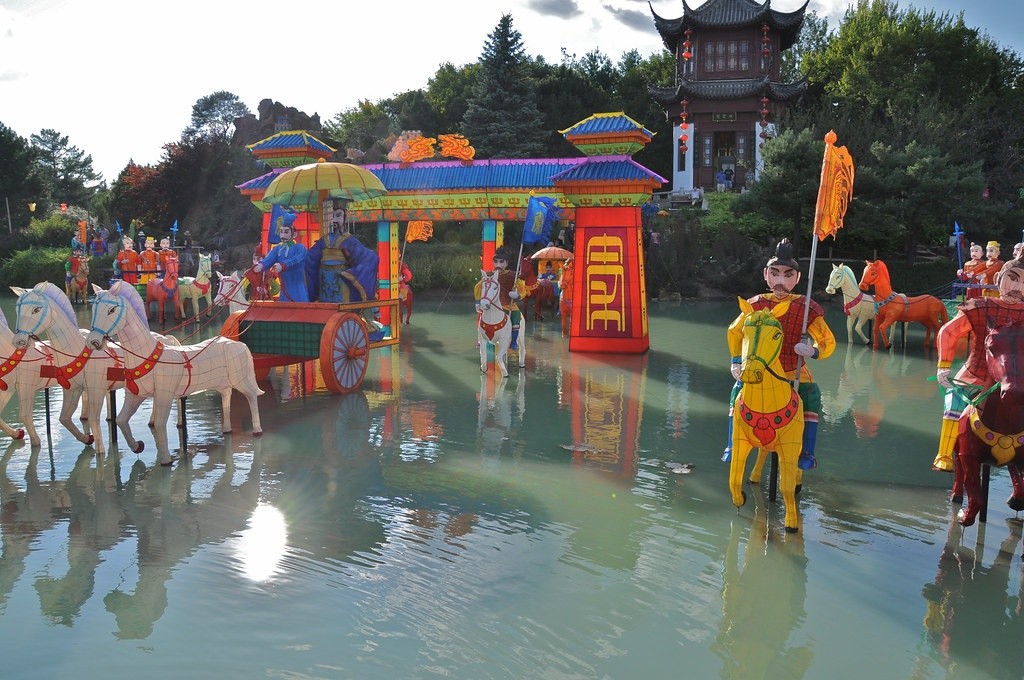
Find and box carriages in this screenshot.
[825,259,1001,353]
[1,252,400,462]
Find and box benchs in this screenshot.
[153,239,203,256]
[670,195,702,208]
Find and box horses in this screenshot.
[729,296,804,533]
[66,252,250,325]
[826,259,949,348]
[478,268,525,378]
[949,316,1024,526]
[0,280,265,466]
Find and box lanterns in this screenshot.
[681,27,692,60]
[680,101,688,154]
[761,25,770,57]
[760,97,769,147]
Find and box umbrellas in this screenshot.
[261,158,389,238]
[531,245,573,260]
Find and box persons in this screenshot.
[138,231,146,252]
[86,224,109,255]
[474,245,527,350]
[540,260,556,280]
[720,238,837,471]
[118,235,176,282]
[724,166,734,188]
[65,230,83,295]
[399,244,412,302]
[184,231,194,264]
[255,196,379,302]
[931,241,1024,472]
[691,186,701,205]
[716,168,726,194]
